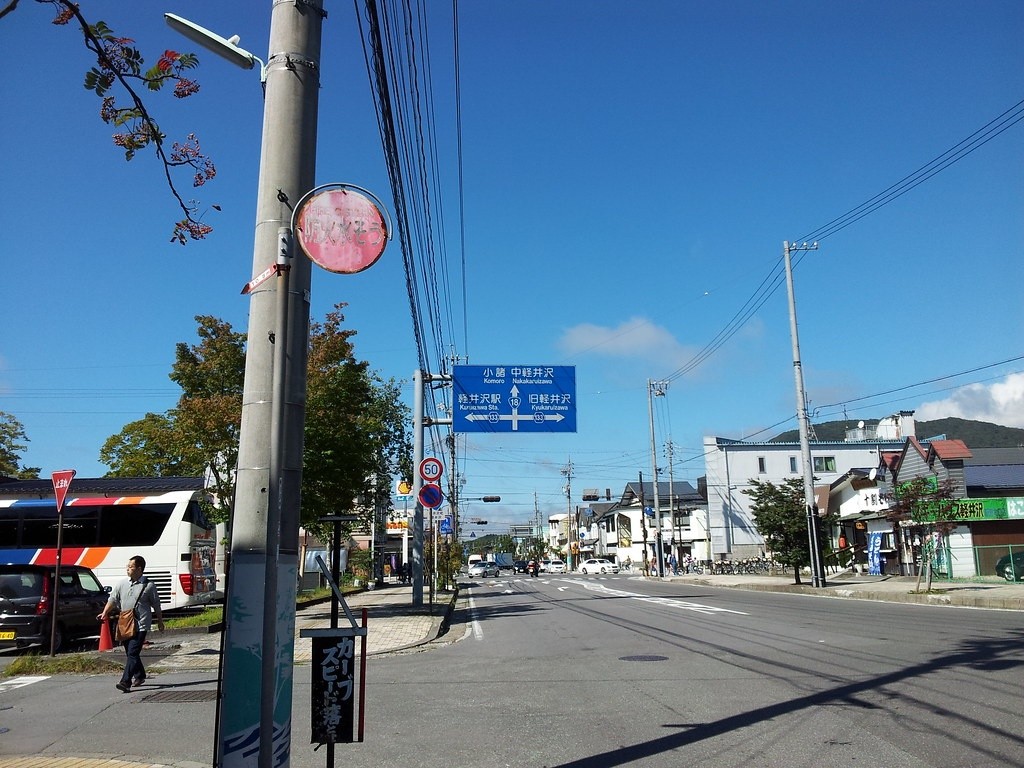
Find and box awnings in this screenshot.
[833,511,892,520]
[561,542,579,554]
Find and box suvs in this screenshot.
[513,561,528,575]
[0,564,121,653]
[468,562,499,578]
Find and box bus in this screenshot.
[0,491,216,617]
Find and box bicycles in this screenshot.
[693,558,784,575]
[665,564,685,576]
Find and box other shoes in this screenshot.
[133,677,146,687]
[116,682,131,693]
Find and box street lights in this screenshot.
[163,11,320,768]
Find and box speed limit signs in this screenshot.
[419,457,443,481]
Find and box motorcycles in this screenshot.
[528,565,540,577]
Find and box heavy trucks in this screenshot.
[495,553,513,570]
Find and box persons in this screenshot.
[625,555,631,569]
[642,550,657,576]
[96,556,164,693]
[528,558,539,573]
[667,549,691,574]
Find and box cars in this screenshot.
[539,560,566,574]
[579,558,620,575]
[995,550,1024,581]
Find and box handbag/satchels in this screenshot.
[114,609,136,641]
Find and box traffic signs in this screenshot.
[452,365,577,433]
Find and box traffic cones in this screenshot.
[98,614,113,652]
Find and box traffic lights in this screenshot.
[477,521,487,525]
[548,520,559,523]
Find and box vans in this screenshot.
[468,555,482,571]
